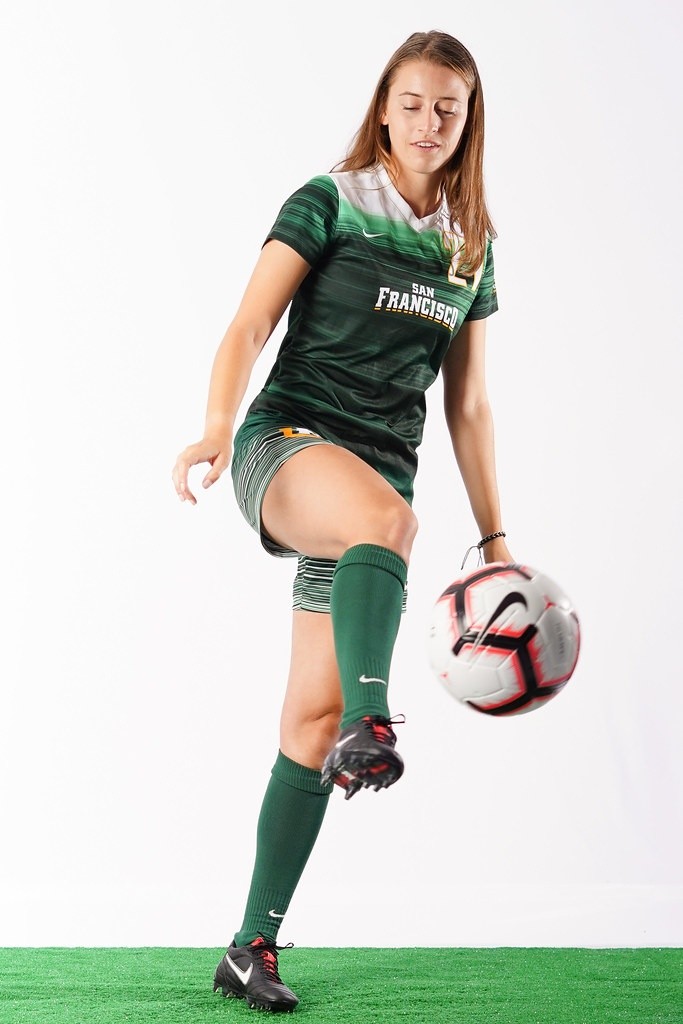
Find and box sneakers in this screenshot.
[321,715,404,799]
[212,938,299,1013]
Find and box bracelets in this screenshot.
[460,531,507,572]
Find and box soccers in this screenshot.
[421,561,580,717]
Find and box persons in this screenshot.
[174,29,516,1011]
